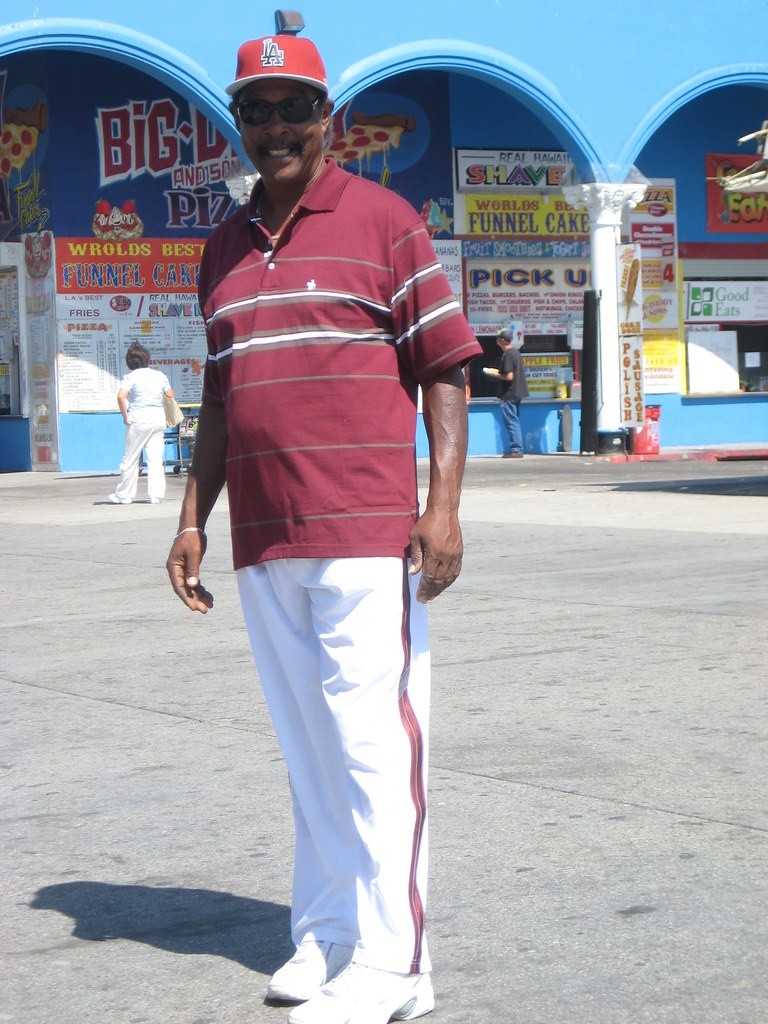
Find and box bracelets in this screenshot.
[173,527,207,538]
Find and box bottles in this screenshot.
[555,380,568,398]
[569,380,581,399]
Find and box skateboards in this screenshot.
[558,402,574,453]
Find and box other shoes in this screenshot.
[151,497,160,504]
[109,494,132,504]
[503,452,523,458]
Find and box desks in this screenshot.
[137,403,200,477]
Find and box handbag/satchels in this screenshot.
[157,373,184,428]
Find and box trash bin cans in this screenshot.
[627,404,662,455]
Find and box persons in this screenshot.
[109,342,174,503]
[167,34,486,1023]
[485,330,531,457]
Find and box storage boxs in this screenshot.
[621,421,660,455]
[596,428,626,455]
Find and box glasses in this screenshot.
[237,96,321,125]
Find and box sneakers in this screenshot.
[267,938,356,1001]
[288,960,434,1024]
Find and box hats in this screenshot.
[225,34,328,94]
